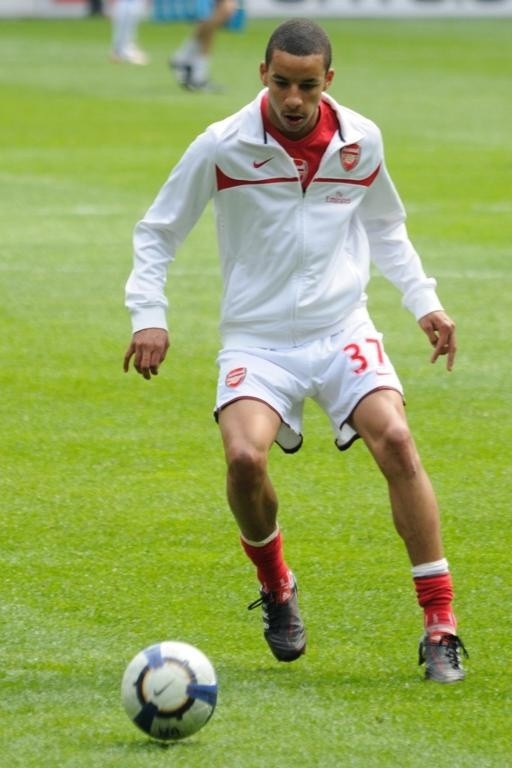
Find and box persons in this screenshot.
[118,16,472,685]
[107,0,153,66]
[161,0,242,93]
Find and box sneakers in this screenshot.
[247,568,307,665]
[420,630,469,684]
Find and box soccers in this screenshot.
[121,642,217,740]
